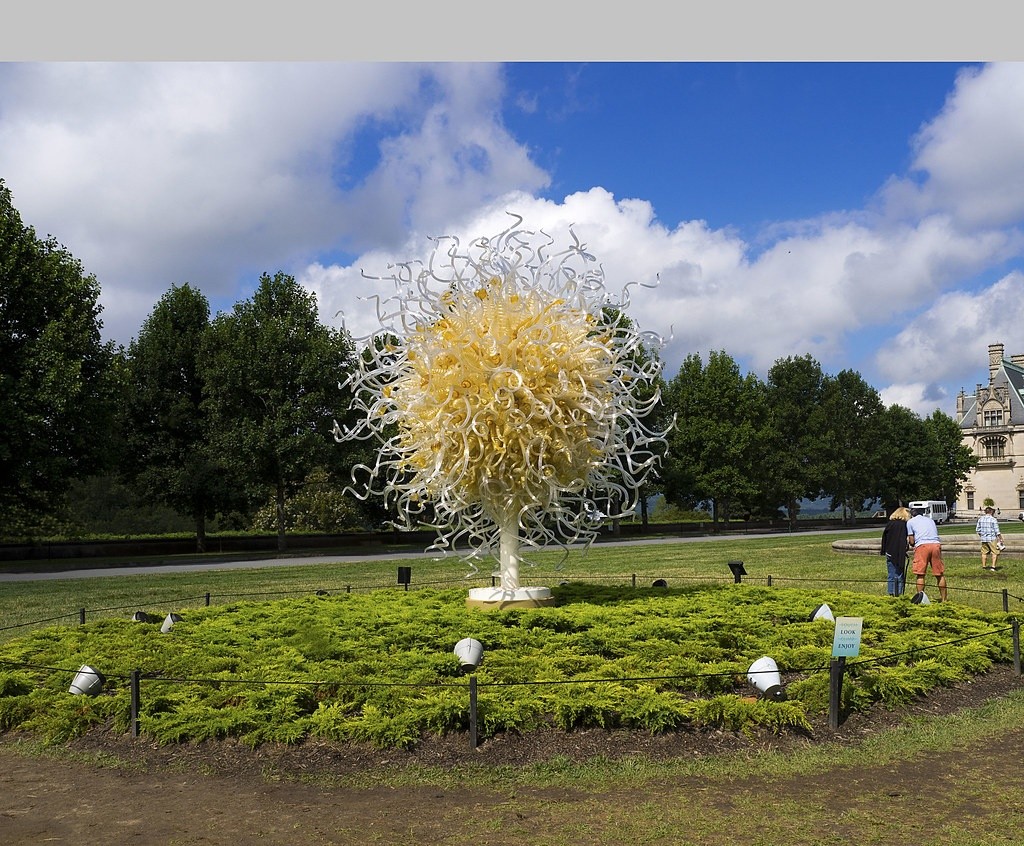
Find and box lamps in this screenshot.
[454,638,483,670]
[808,603,836,625]
[69,663,107,697]
[132,612,147,623]
[160,612,185,634]
[651,579,670,590]
[745,656,787,703]
[911,591,932,608]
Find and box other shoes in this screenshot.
[990,566,998,571]
[982,567,986,569]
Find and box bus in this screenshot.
[909,501,948,525]
